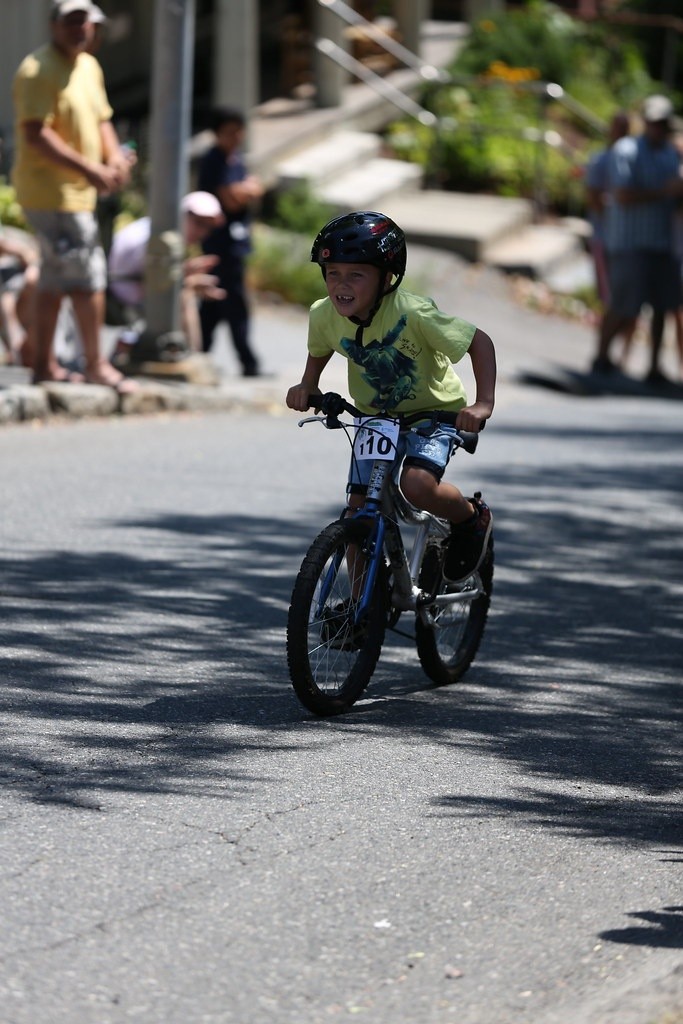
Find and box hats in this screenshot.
[644,95,672,123]
[48,0,107,24]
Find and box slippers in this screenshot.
[85,365,137,391]
[33,368,86,382]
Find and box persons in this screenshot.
[13,0,129,397]
[585,94,683,386]
[0,106,277,374]
[286,212,496,648]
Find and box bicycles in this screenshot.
[285,391,498,719]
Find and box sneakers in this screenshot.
[440,491,493,583]
[319,597,367,647]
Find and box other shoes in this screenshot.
[111,339,135,372]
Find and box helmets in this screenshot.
[310,210,407,276]
[179,191,222,218]
